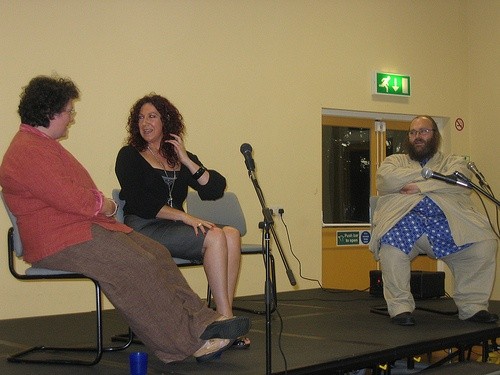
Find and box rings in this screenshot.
[198,223,202,226]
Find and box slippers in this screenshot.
[200,317,249,340]
[196,338,235,363]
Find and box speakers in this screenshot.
[369,270,445,298]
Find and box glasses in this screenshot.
[63,109,76,116]
[408,129,434,136]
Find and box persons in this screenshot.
[114,92,253,350]
[0,74,251,366]
[368,115,500,326]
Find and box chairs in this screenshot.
[0,190,277,366]
[369,196,458,317]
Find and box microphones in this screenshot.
[422,167,473,189]
[467,162,488,185]
[240,143,255,171]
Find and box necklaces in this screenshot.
[146,146,176,208]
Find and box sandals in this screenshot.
[230,338,250,349]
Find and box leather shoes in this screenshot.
[467,310,498,323]
[391,312,417,325]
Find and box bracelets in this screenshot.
[106,198,118,218]
[192,166,206,180]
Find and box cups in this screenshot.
[129,351,148,375]
